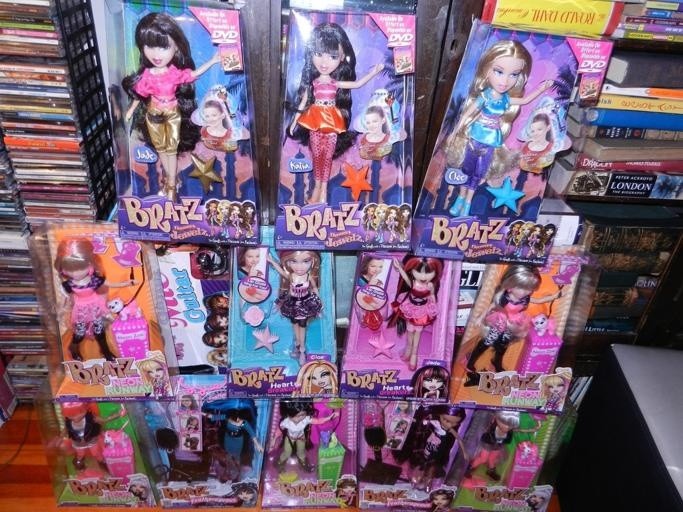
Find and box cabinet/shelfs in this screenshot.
[0,1,116,408]
[554,1,683,385]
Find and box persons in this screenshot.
[26,0,625,512]
